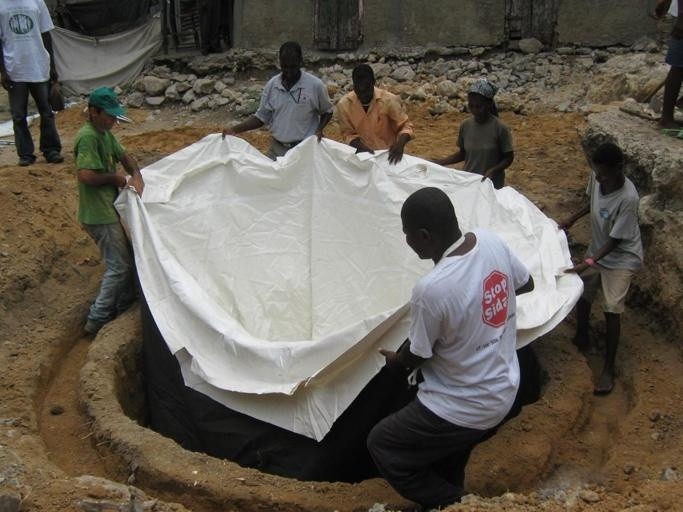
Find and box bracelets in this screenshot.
[582,257,598,269]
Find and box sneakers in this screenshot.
[44,148,64,162]
[20,155,33,165]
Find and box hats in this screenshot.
[89,87,132,124]
[469,80,498,99]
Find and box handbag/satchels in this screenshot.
[51,81,66,111]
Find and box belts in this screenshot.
[276,139,300,147]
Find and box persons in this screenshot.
[336,66,416,168]
[72,86,145,337]
[0,0,66,167]
[368,186,538,512]
[650,1,682,133]
[430,79,515,191]
[222,41,334,160]
[556,143,647,395]
